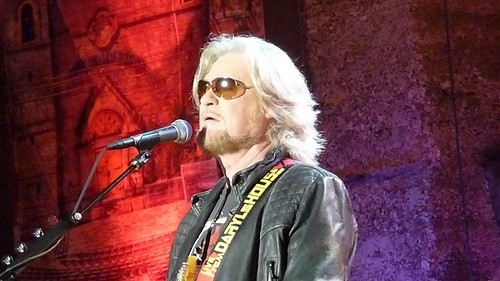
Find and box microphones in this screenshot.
[106,119,193,150]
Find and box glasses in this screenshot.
[197,77,253,100]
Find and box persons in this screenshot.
[166,34,357,281]
